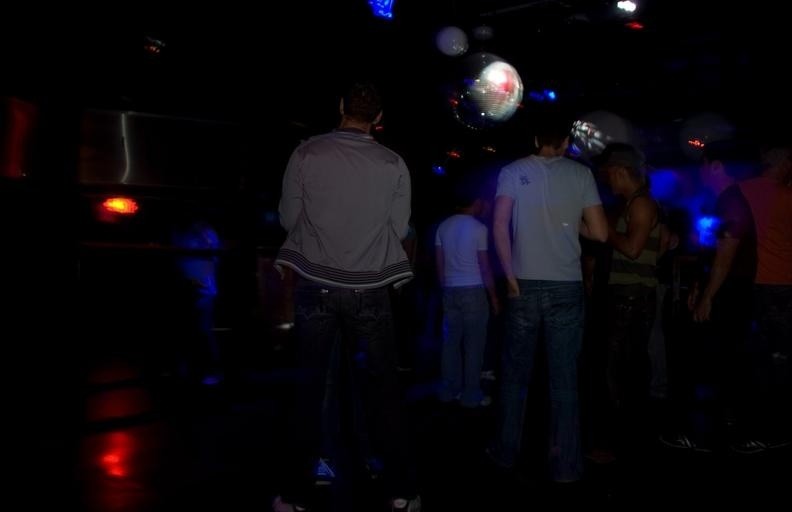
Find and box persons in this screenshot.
[274,77,791,509]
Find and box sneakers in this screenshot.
[667,432,716,450]
[745,437,783,454]
[393,497,422,511]
